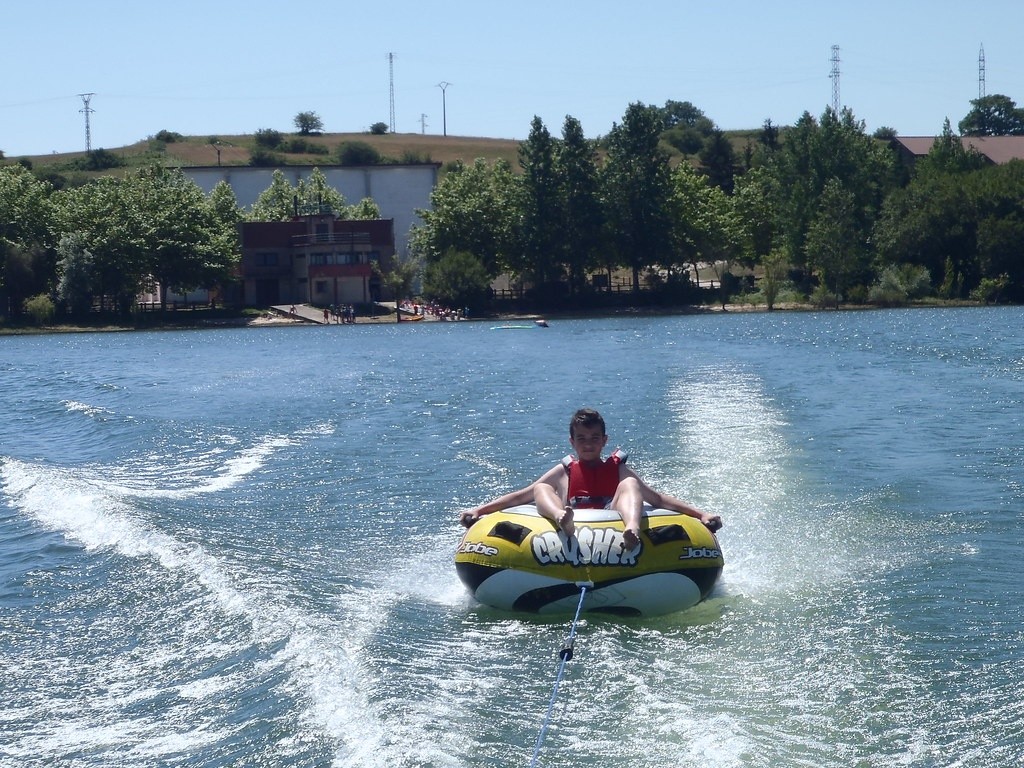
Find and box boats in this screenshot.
[400,314,425,321]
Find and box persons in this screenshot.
[459,409,723,551]
[398,297,472,321]
[289,303,297,313]
[323,304,356,324]
